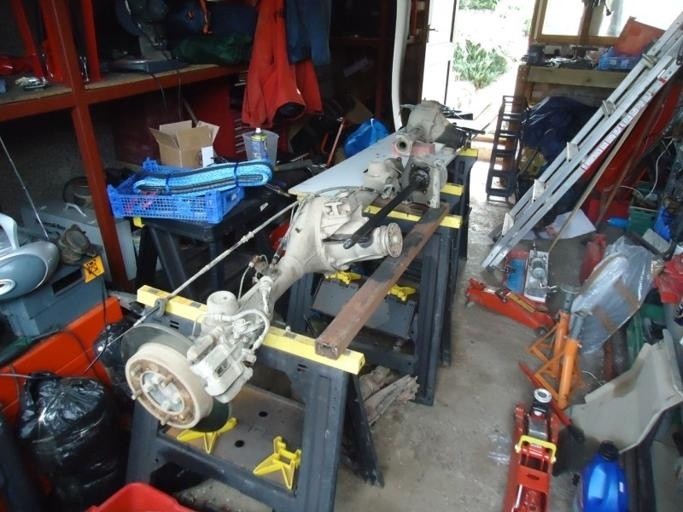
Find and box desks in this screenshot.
[527,65,627,88]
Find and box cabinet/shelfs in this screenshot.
[0,1,289,293]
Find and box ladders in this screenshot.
[480,11,683,273]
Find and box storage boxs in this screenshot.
[148,119,219,170]
[107,158,244,225]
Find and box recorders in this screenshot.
[1,140,59,302]
